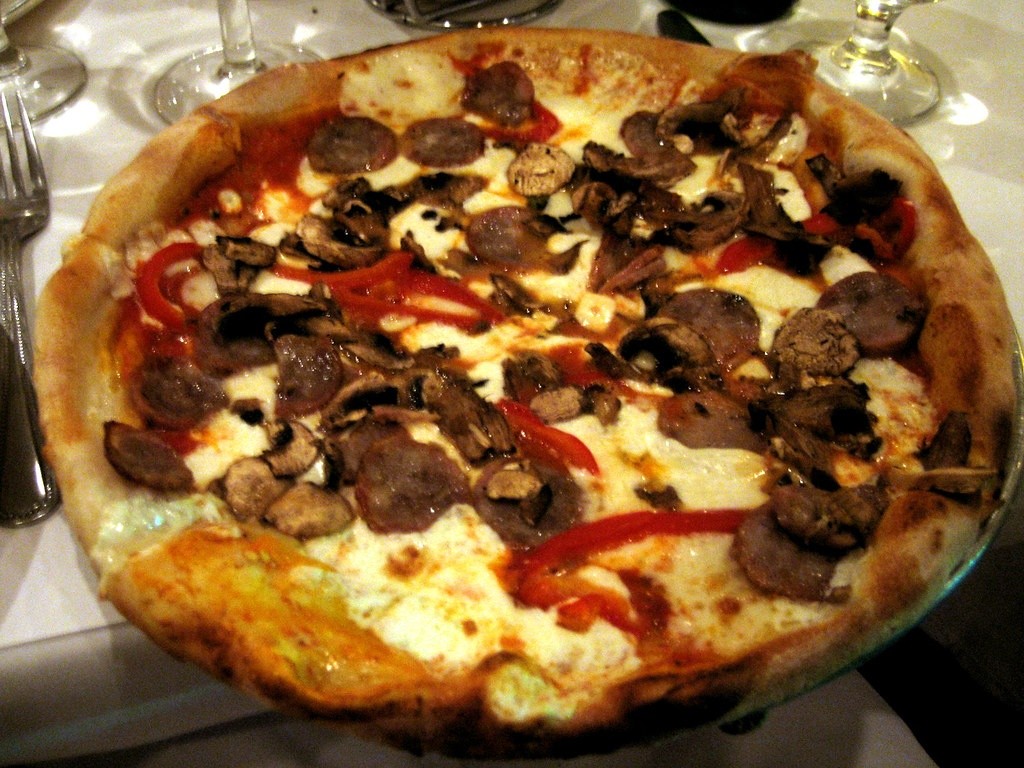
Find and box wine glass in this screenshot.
[783,0,942,127]
[153,0,327,128]
[0,0,89,135]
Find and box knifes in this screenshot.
[654,9,716,49]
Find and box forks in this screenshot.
[0,89,63,528]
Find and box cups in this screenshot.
[657,0,799,26]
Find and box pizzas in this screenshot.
[32,24,1014,745]
